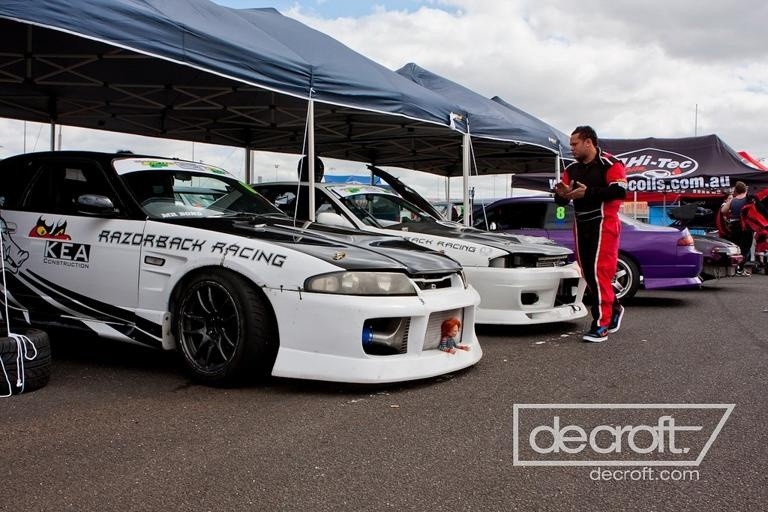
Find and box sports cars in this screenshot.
[1,151,588,388]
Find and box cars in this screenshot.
[402,194,742,307]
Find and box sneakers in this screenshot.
[582,326,608,342]
[736,267,751,277]
[608,304,625,333]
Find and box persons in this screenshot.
[436,317,473,354]
[551,122,630,343]
[720,182,754,277]
[714,193,735,242]
[753,228,768,266]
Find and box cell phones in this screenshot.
[555,182,568,189]
